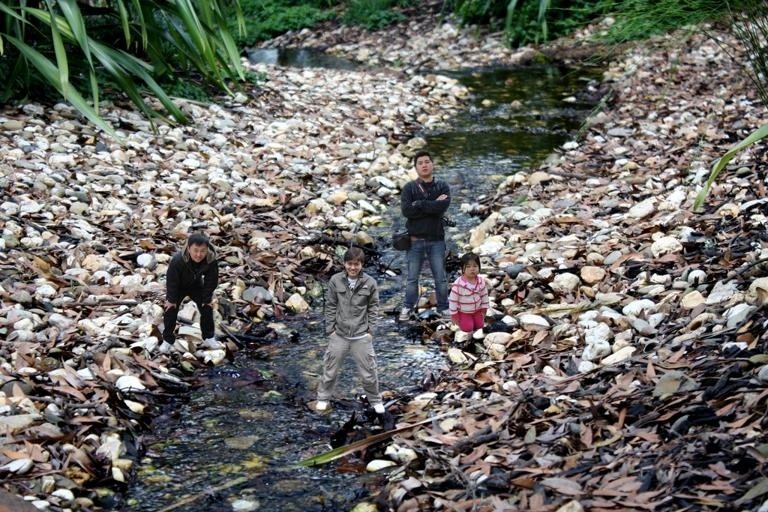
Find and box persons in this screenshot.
[398,151,457,321]
[276,38,287,65]
[316,247,385,416]
[240,47,257,65]
[448,252,488,363]
[159,234,225,352]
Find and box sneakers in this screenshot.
[317,400,330,410]
[398,307,414,321]
[159,340,171,352]
[202,338,221,349]
[374,404,386,414]
[441,310,452,319]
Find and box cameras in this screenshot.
[441,215,456,227]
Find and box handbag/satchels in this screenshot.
[392,229,410,251]
